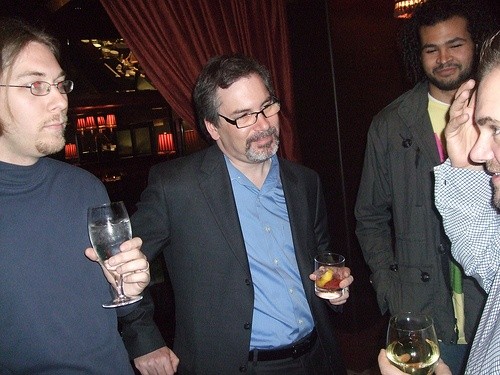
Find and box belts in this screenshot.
[248,326,318,361]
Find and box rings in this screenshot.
[344,289,350,292]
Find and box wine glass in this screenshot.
[87,200,143,308]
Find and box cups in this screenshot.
[385,312,441,375]
[312,252,345,300]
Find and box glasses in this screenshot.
[218,98,281,129]
[1,80,73,96]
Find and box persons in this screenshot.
[0,16,150,375]
[353,0,489,375]
[378,29,500,375]
[117,51,375,375]
